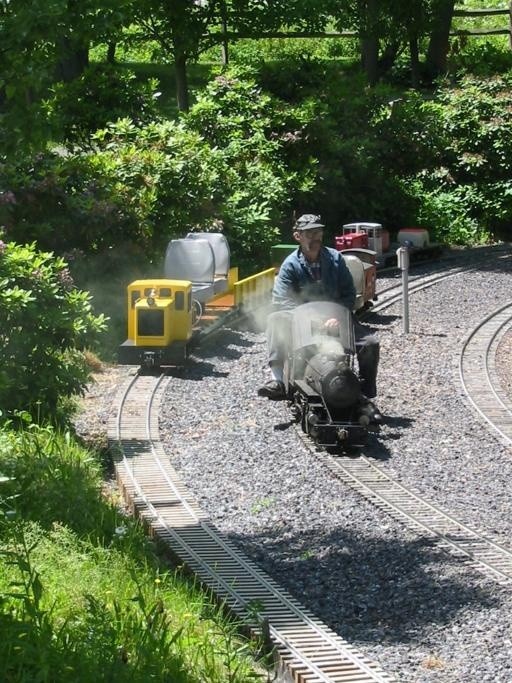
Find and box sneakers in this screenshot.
[258,379,289,401]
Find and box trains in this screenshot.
[120,231,303,375]
[335,222,446,270]
[284,247,429,451]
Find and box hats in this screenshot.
[291,211,327,238]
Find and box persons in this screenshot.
[255,211,383,404]
